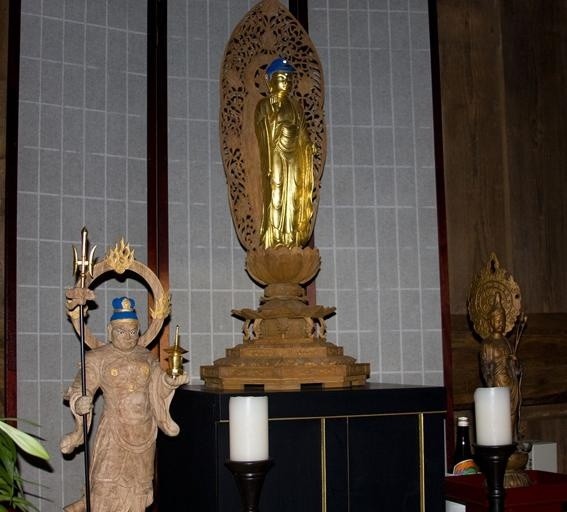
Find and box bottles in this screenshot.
[451,417,478,476]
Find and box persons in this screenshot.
[59,298,190,512]
[480,292,523,443]
[254,58,317,250]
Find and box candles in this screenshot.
[227,396,269,461]
[473,386,514,446]
